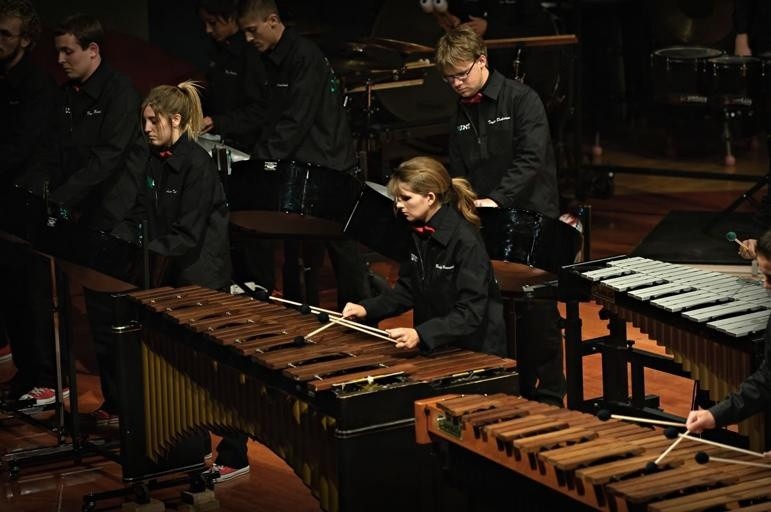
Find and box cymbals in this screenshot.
[336,40,396,60]
[371,35,437,54]
[337,62,395,86]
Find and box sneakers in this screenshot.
[19,385,119,426]
[201,463,249,484]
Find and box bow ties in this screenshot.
[159,151,174,159]
[461,93,483,107]
[415,226,435,236]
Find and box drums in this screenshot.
[653,46,729,96]
[709,55,767,98]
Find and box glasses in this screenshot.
[441,56,478,84]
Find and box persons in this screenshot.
[686,230,771,458]
[738,239,758,260]
[733,3,770,56]
